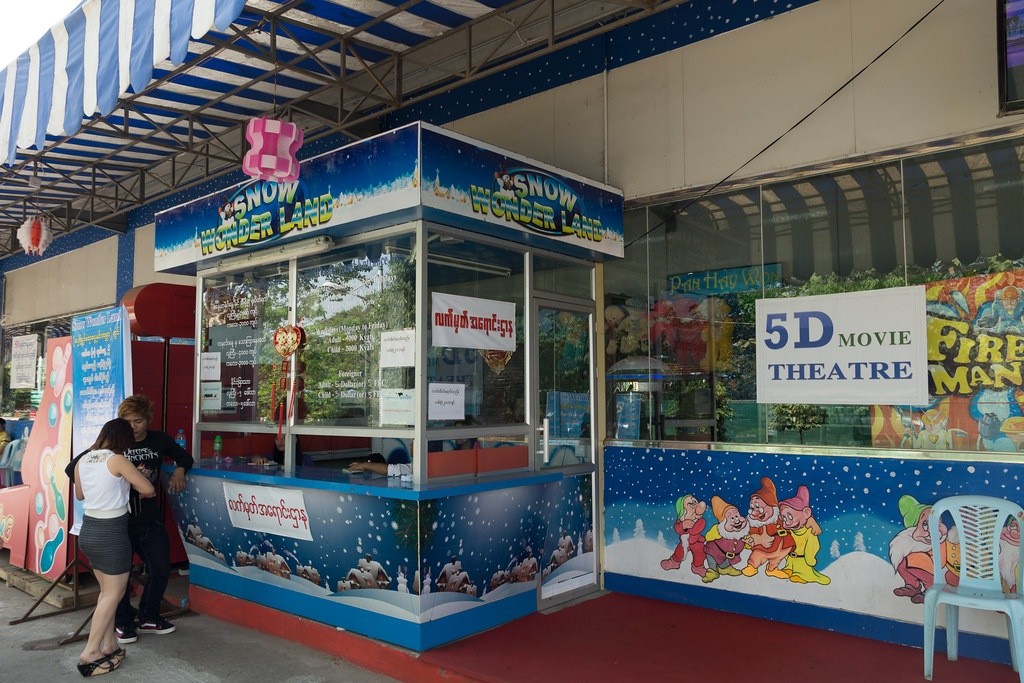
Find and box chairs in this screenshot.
[924,495,1024,683]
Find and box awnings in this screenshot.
[0,0,519,230]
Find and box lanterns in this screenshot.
[242,115,304,183]
[16,216,53,256]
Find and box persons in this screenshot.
[453,415,482,450]
[348,439,413,476]
[251,433,315,466]
[73,417,157,678]
[115,394,195,644]
[0,418,11,454]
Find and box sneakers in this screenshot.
[114,625,137,643]
[139,618,176,635]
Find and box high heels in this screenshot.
[77,655,120,678]
[105,644,125,661]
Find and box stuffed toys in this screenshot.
[697,297,734,372]
[556,310,587,377]
[672,298,708,363]
[644,298,678,349]
[618,298,653,352]
[604,305,631,353]
